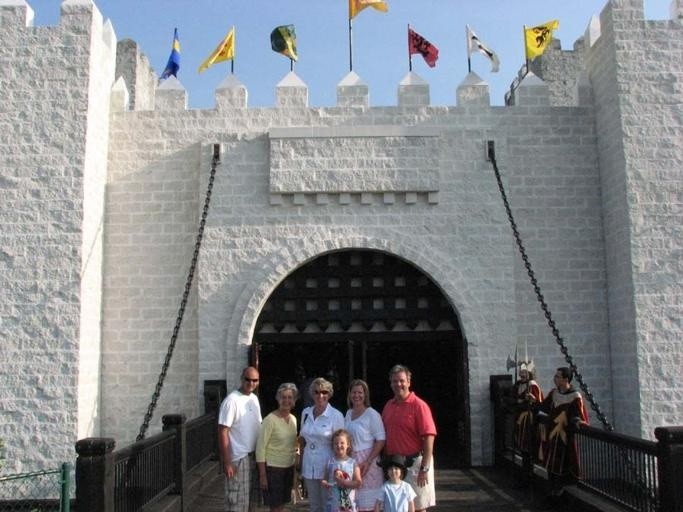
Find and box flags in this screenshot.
[159,28,180,80]
[466,24,499,72]
[270,25,298,62]
[406,25,437,68]
[524,21,558,61]
[349,1,386,18]
[197,28,233,72]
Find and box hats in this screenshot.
[382,455,408,481]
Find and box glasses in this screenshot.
[244,376,258,382]
[315,390,329,395]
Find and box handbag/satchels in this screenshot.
[294,436,306,471]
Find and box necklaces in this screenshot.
[241,393,253,412]
[390,481,403,490]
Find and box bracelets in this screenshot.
[366,457,372,464]
[420,464,429,472]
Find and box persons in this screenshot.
[508,362,543,489]
[380,365,437,511]
[373,462,417,511]
[343,379,386,511]
[255,382,300,511]
[319,428,361,512]
[295,376,344,511]
[217,366,264,511]
[526,367,590,504]
